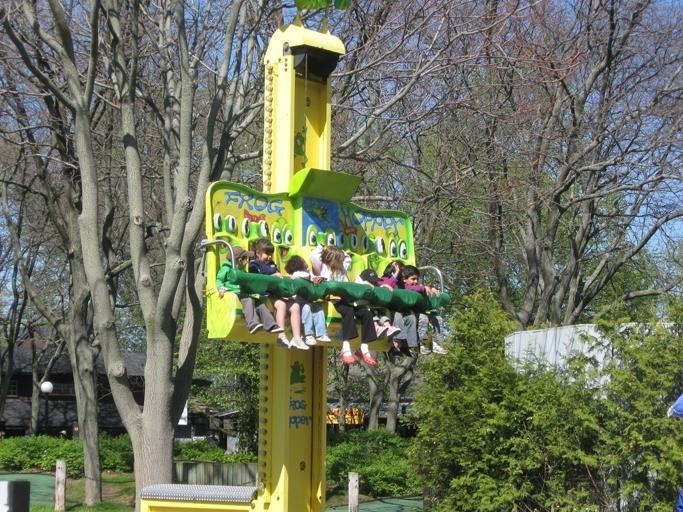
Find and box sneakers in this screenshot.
[340,349,355,364]
[278,334,290,346]
[290,337,310,350]
[305,335,317,345]
[360,349,376,365]
[250,324,263,334]
[376,326,388,338]
[316,336,331,342]
[270,325,285,333]
[432,345,448,354]
[419,345,431,355]
[387,327,401,337]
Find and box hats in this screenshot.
[226,246,255,261]
[361,269,381,282]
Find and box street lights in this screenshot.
[40,381,54,435]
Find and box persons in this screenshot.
[216,238,449,366]
